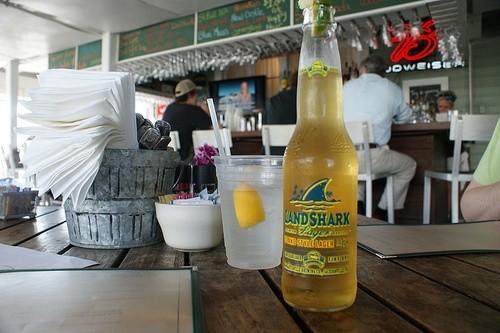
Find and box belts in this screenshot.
[354,144,377,151]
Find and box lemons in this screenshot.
[233,182,264,228]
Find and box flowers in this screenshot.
[191,142,219,166]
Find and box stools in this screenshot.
[168,127,233,157]
[423,113,500,224]
[343,118,395,225]
[260,125,297,156]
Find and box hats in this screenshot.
[175,79,203,98]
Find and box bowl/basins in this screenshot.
[154,200,222,251]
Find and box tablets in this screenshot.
[356,220,500,261]
[0,266,205,333]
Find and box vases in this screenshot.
[192,164,217,194]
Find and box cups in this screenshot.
[211,155,283,270]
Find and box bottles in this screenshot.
[281,3,357,313]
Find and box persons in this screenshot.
[343,55,417,222]
[161,79,224,191]
[237,82,253,108]
[435,91,470,222]
[260,71,298,156]
[460,118,500,222]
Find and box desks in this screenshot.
[0,204,500,333]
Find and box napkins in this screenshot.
[14,66,139,210]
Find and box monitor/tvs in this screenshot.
[208,74,266,114]
